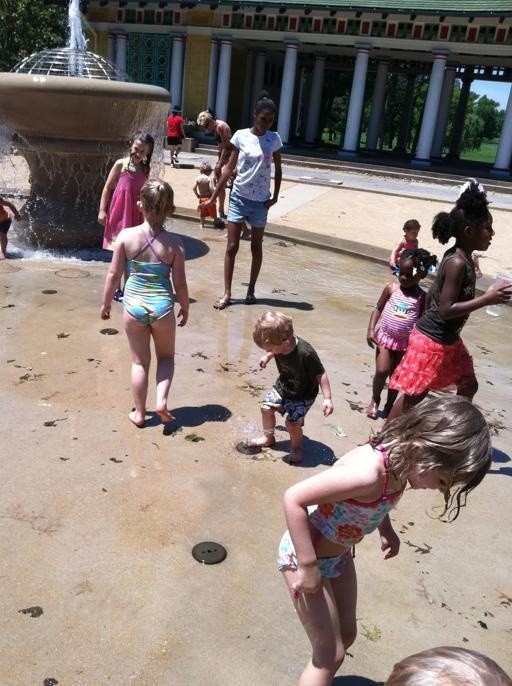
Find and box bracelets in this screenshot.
[368,328,375,330]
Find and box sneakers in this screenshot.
[170,164,174,168]
[171,154,179,163]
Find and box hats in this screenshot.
[171,105,182,112]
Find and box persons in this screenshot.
[246,310,334,462]
[365,247,442,419]
[275,392,493,686]
[386,645,511,686]
[386,177,512,416]
[387,218,422,279]
[95,127,155,304]
[0,195,24,261]
[167,103,187,168]
[99,176,189,428]
[197,109,234,220]
[199,89,286,309]
[193,159,217,231]
